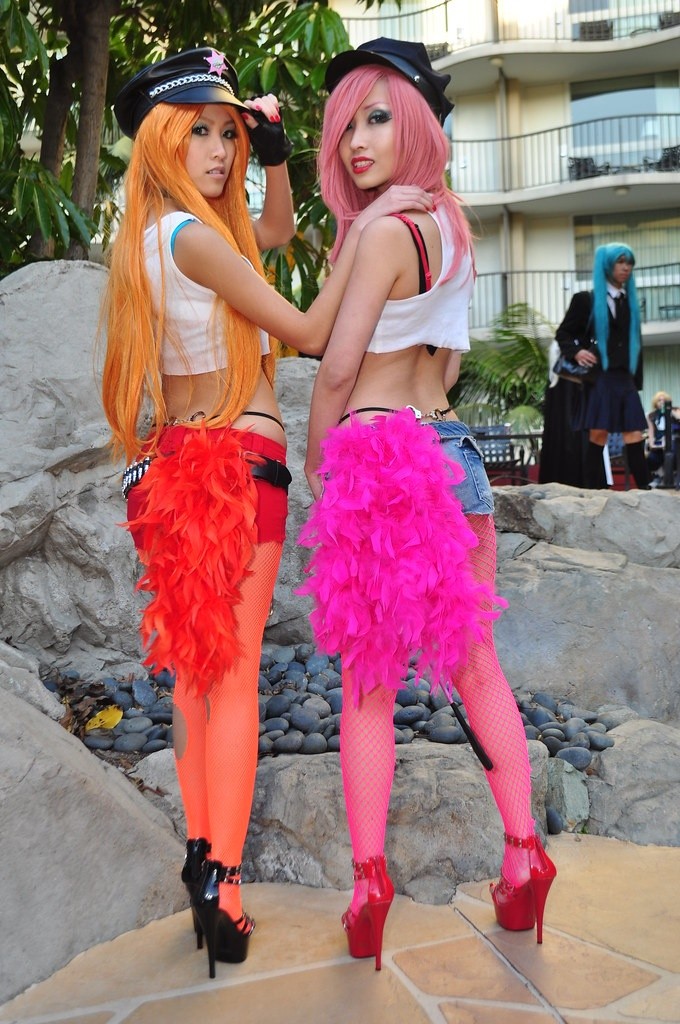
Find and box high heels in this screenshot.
[180,836,213,950]
[342,855,394,972]
[490,834,557,944]
[189,859,256,979]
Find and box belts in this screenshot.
[121,457,292,496]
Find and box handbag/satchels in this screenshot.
[553,293,599,385]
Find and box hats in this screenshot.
[326,37,454,128]
[114,47,253,140]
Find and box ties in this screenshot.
[609,291,625,320]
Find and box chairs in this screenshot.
[659,12,680,30]
[643,145,680,173]
[579,20,614,41]
[568,156,597,181]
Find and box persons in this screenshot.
[303,38,556,971]
[553,241,651,491]
[100,44,435,980]
[644,387,680,475]
